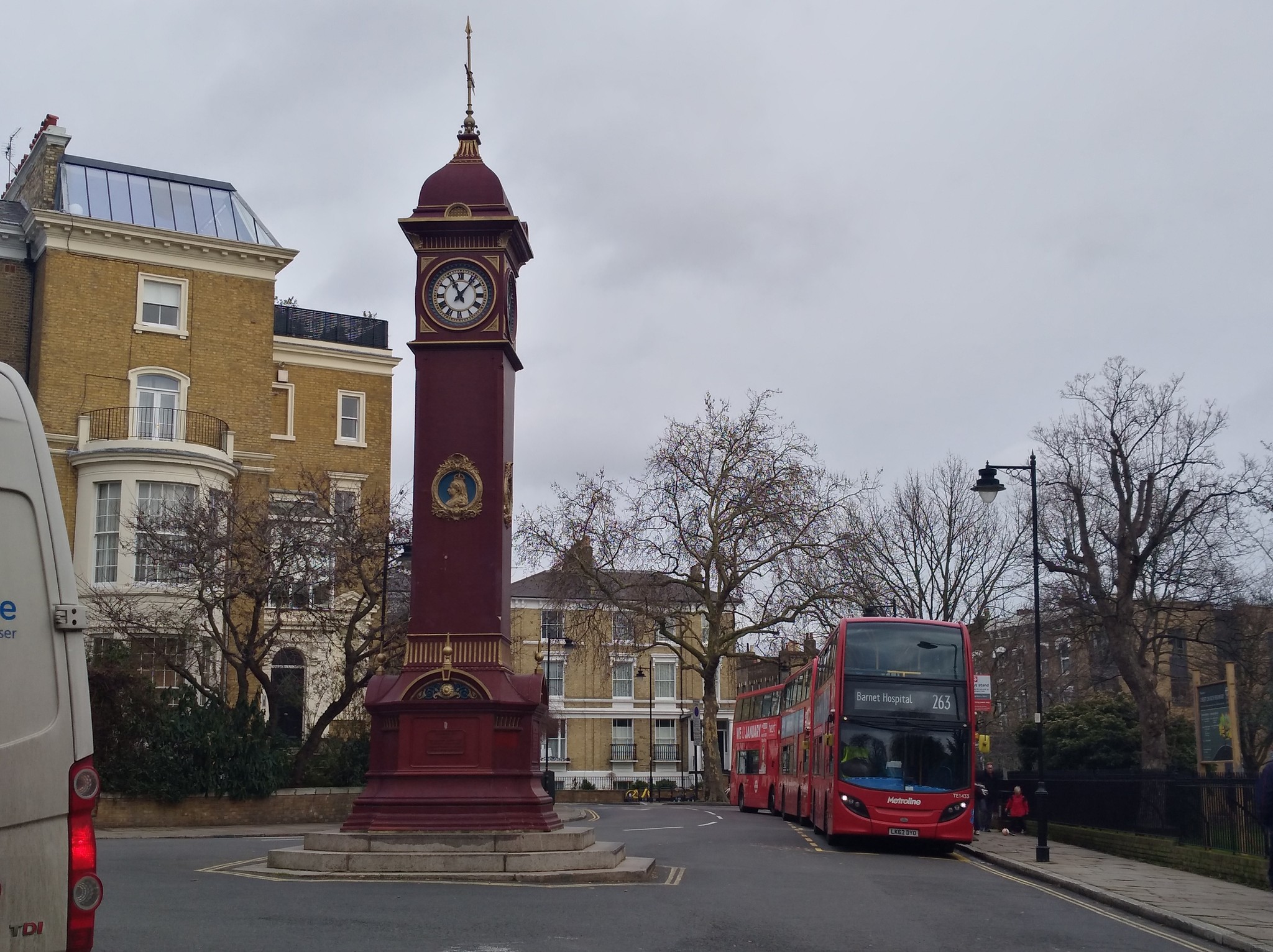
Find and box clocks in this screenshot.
[427,262,492,326]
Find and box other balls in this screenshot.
[1002,828,1009,836]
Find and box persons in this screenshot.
[1004,786,1030,834]
[974,760,999,836]
[445,479,469,508]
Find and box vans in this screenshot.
[0,361,106,952]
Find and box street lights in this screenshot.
[969,450,1052,863]
[633,659,653,803]
[543,633,575,790]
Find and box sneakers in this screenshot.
[1010,831,1016,836]
[973,830,980,835]
[984,829,990,832]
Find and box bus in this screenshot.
[775,616,993,858]
[728,682,783,816]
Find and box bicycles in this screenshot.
[624,784,651,803]
